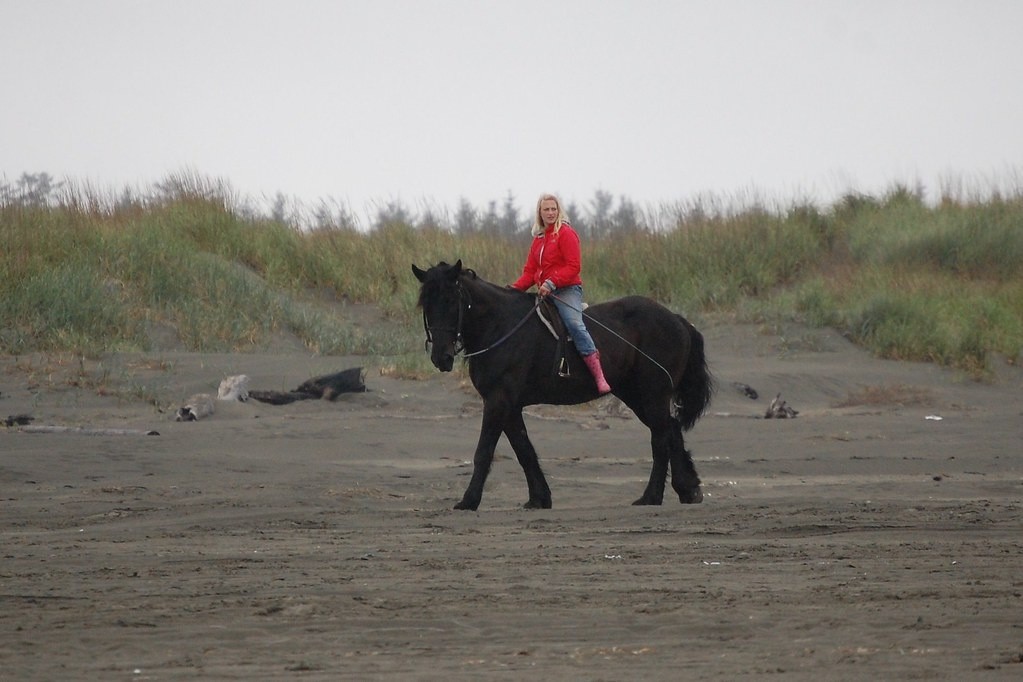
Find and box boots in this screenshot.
[583,350,611,396]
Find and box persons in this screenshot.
[509,194,611,395]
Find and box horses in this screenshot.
[411,259,712,510]
